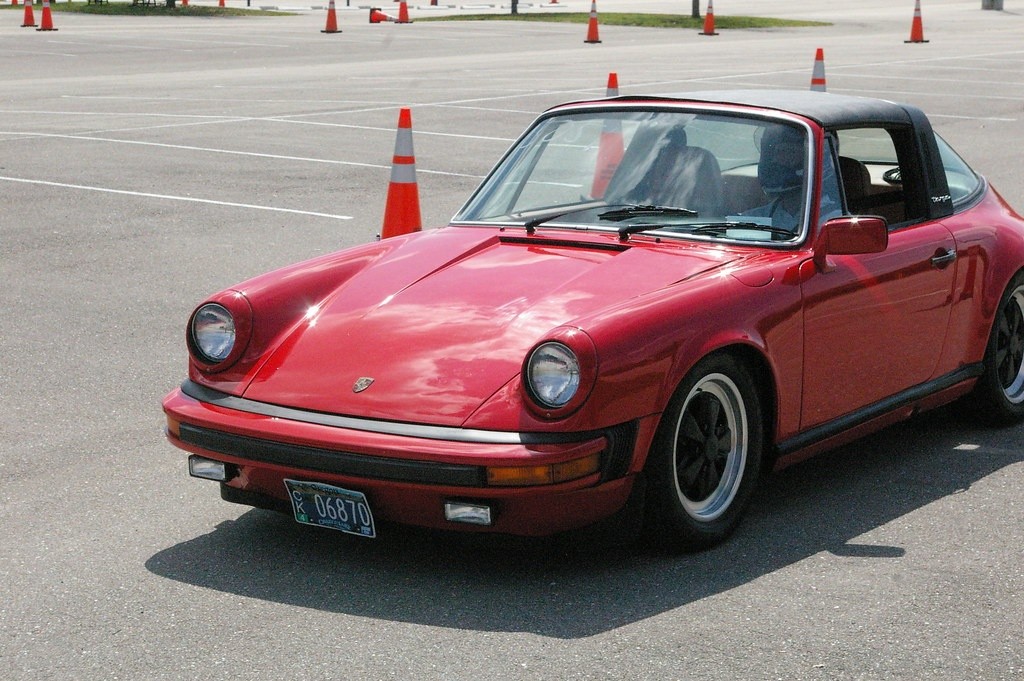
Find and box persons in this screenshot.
[733,125,852,236]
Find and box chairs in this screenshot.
[611,147,727,214]
[836,159,875,220]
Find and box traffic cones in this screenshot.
[429,0,438,6]
[576,72,627,203]
[394,1,414,24]
[319,0,342,33]
[369,7,399,24]
[11,0,19,6]
[549,0,559,4]
[376,106,424,241]
[181,0,189,6]
[809,46,829,92]
[698,0,720,35]
[583,0,602,44]
[903,0,930,44]
[35,0,59,32]
[217,0,225,7]
[21,0,39,28]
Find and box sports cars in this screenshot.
[161,86,1024,554]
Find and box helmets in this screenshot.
[757,124,840,201]
